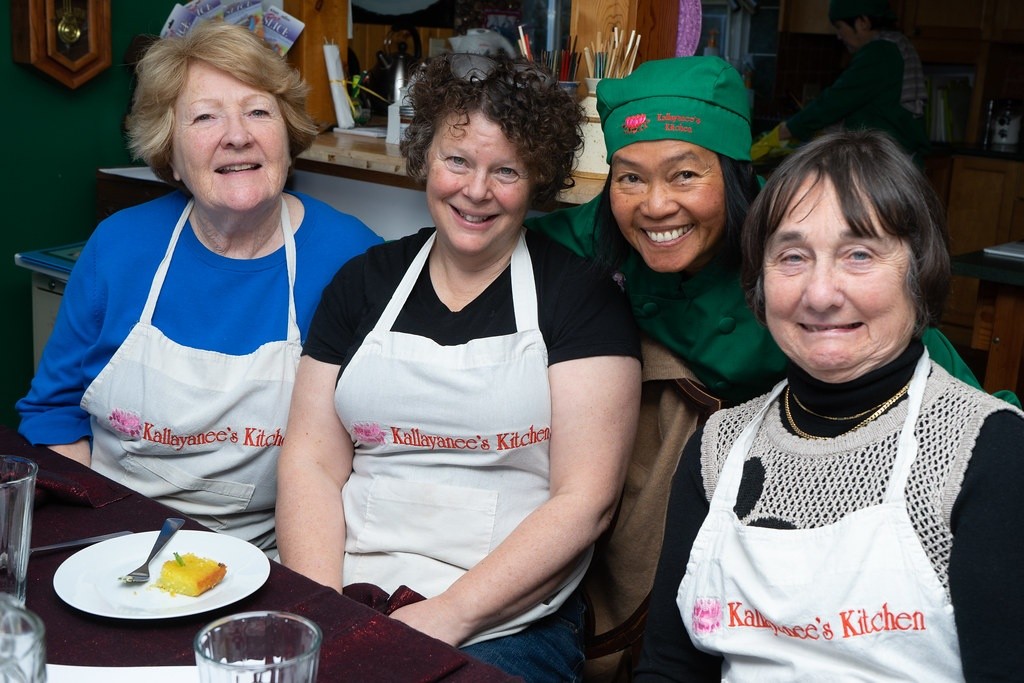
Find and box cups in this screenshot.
[192,609,323,683]
[1,593,48,683]
[0,453,40,611]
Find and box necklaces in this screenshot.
[785,378,911,440]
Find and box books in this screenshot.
[19,241,88,274]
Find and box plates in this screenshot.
[54,529,272,619]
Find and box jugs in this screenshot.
[984,96,1024,153]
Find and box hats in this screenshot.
[596,54,754,163]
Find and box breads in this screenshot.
[156,551,227,596]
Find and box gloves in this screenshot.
[749,123,790,162]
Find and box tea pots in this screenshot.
[368,19,422,116]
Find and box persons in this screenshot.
[16,20,384,558]
[748,1,935,176]
[632,130,1024,683]
[523,57,1022,411]
[274,50,644,683]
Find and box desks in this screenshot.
[0,422,528,683]
[947,250,1024,394]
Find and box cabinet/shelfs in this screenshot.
[777,0,1024,45]
[14,243,89,376]
[922,155,1024,330]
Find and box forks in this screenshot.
[118,516,185,583]
